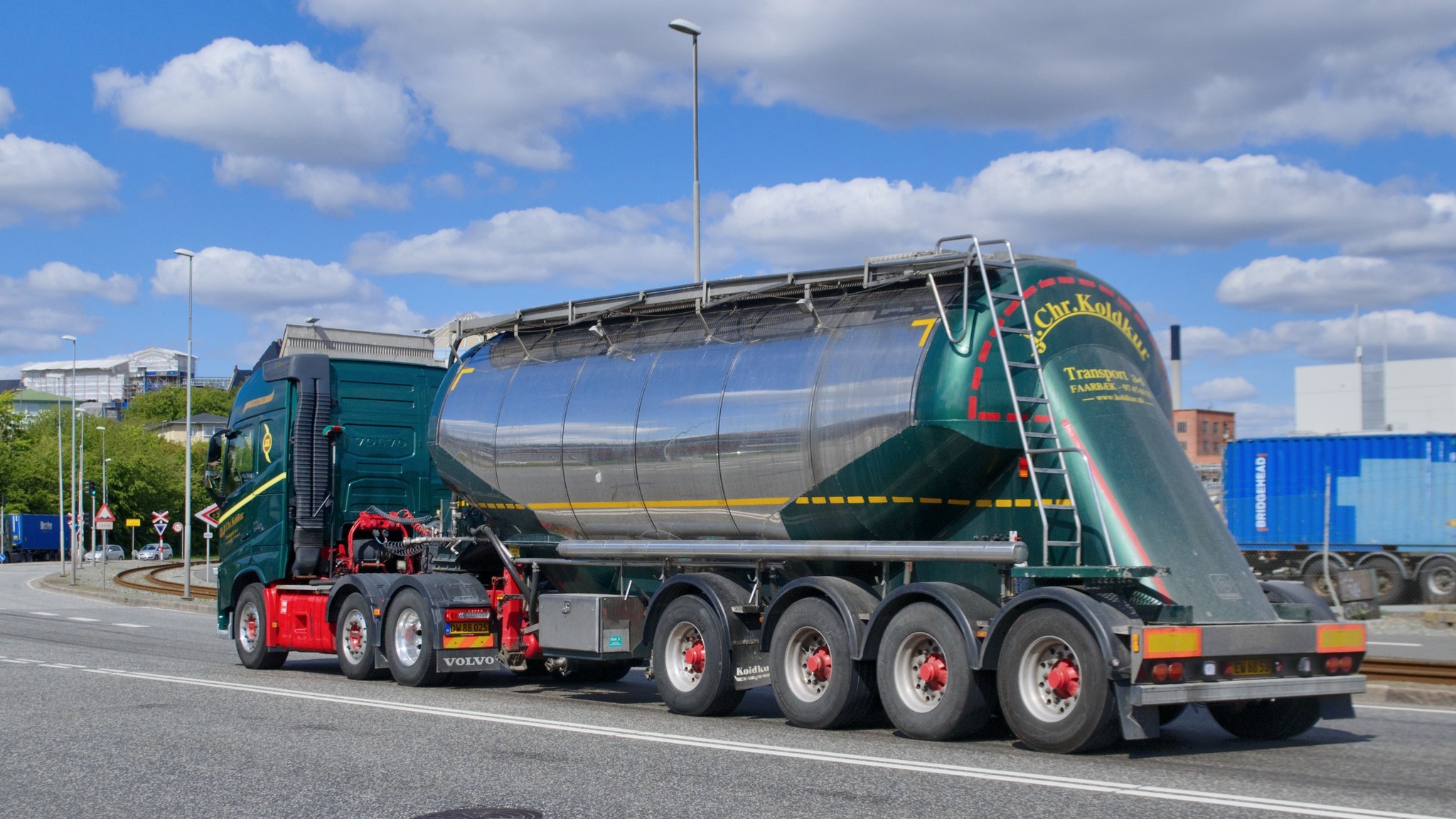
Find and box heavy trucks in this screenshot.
[205,232,1372,755]
[1216,430,1456,609]
[0,512,78,564]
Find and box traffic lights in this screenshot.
[89,480,96,496]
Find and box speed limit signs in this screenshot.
[172,522,182,533]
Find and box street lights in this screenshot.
[96,426,106,565]
[666,17,701,283]
[173,248,195,602]
[60,332,78,586]
[46,372,68,577]
[74,407,86,570]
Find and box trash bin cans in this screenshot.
[1336,563,1379,620]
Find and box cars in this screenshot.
[137,543,173,561]
[84,544,125,563]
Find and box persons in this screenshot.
[208,459,220,467]
